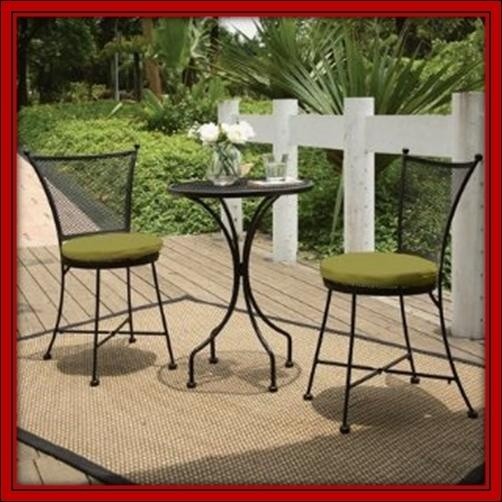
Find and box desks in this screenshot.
[166,173,316,394]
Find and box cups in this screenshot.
[262,152,289,181]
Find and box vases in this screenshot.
[207,141,244,186]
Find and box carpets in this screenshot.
[16,295,485,485]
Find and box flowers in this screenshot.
[196,118,254,178]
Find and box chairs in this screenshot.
[303,146,482,436]
[24,143,177,390]
